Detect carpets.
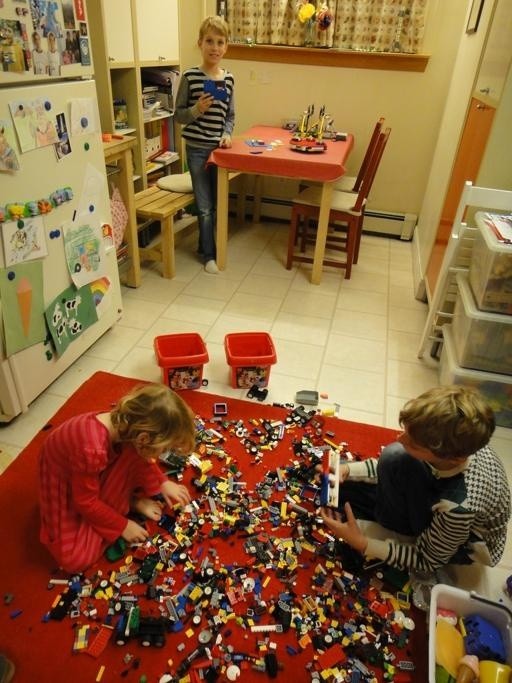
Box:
[2,369,430,683]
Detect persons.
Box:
[30,30,84,76]
[171,13,237,273]
[321,382,511,610]
[34,380,197,571]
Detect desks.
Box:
[205,123,355,286]
[104,133,144,288]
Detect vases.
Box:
[305,20,325,48]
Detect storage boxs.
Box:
[224,331,279,387]
[153,331,210,389]
[470,209,512,313]
[436,320,512,429]
[448,268,512,377]
[426,583,512,683]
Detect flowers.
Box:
[295,0,336,30]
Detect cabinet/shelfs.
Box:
[104,0,183,66]
[414,0,511,339]
[107,63,188,250]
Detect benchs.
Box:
[133,164,249,281]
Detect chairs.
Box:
[292,116,387,255]
[415,180,511,362]
[284,125,393,280]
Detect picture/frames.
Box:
[464,0,485,35]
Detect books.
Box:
[115,238,132,267]
[140,66,180,187]
[105,165,121,177]
